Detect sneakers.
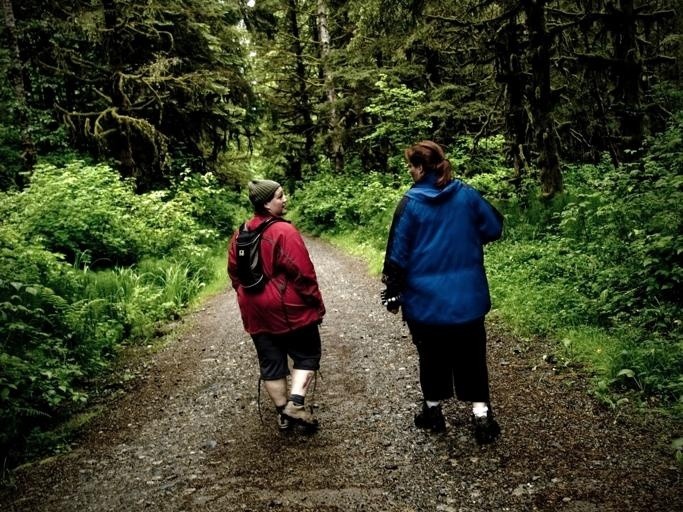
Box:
[276,401,318,431]
[414,399,446,432]
[470,413,499,443]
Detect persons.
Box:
[379,140,505,443]
[224,177,326,435]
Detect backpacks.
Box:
[236,218,274,294]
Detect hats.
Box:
[248,179,280,204]
